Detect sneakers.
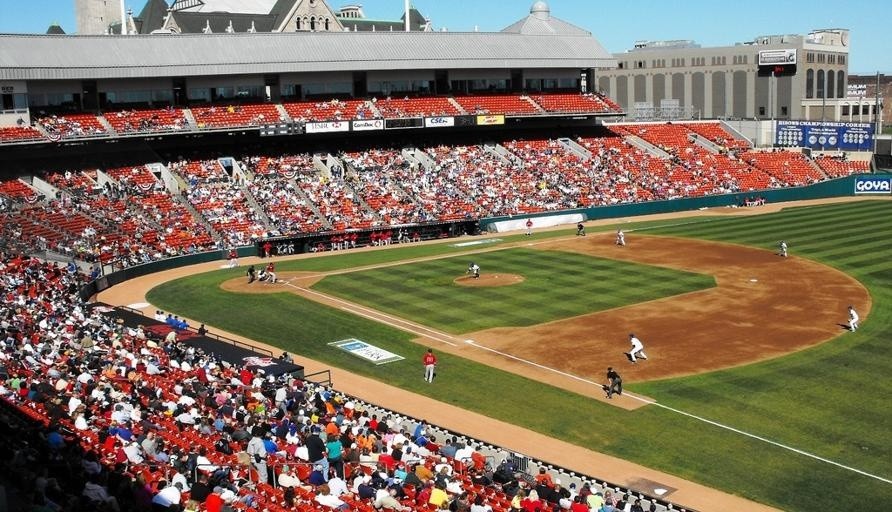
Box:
[424,377,431,383]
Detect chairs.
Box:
[0,108,159,511]
[157,93,850,262]
[161,341,672,512]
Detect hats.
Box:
[428,349,432,352]
[312,427,321,432]
[589,487,597,494]
[175,482,183,489]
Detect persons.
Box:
[266,262,277,285]
[464,261,481,278]
[287,92,875,254]
[775,239,789,260]
[846,306,858,331]
[1,270,214,511]
[626,334,648,364]
[257,267,267,281]
[214,349,656,511]
[1,93,288,269]
[246,263,256,285]
